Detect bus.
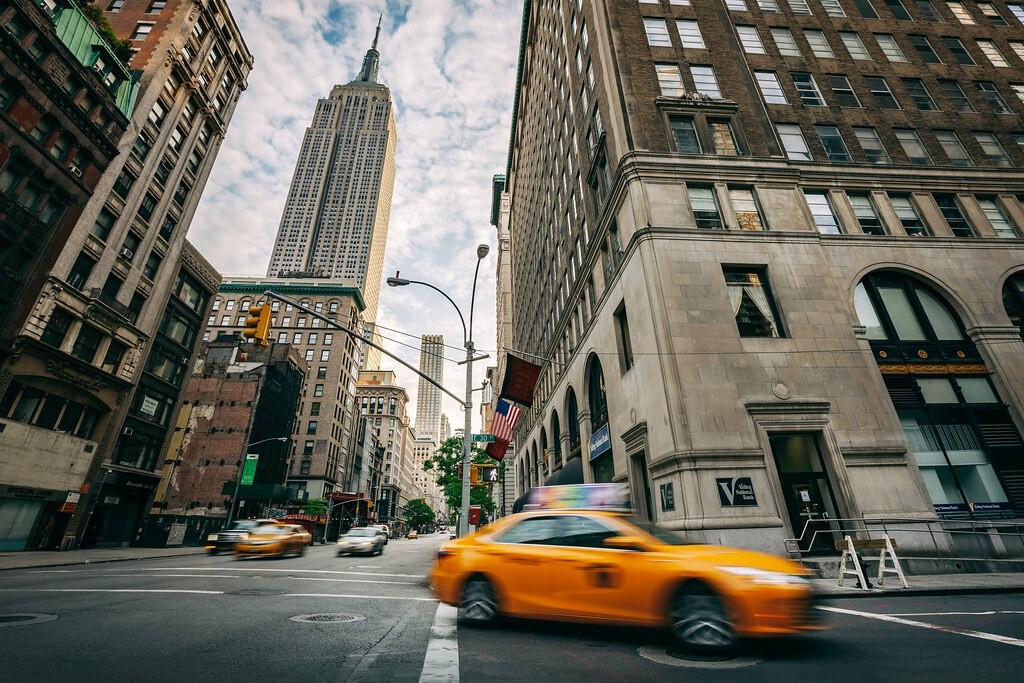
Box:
[438,525,447,535]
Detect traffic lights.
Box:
[368,500,374,508]
[483,465,500,482]
[243,303,270,341]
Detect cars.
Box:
[230,524,313,559]
[449,527,458,539]
[406,529,419,540]
[334,526,385,557]
[367,524,390,545]
[427,482,838,651]
[205,518,279,558]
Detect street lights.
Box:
[226,436,288,529]
[386,242,494,541]
